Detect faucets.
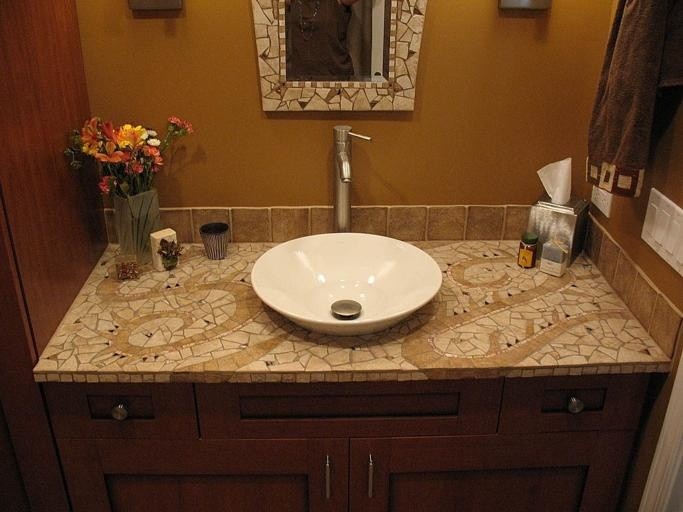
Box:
[331,123,370,232]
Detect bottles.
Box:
[517,232,539,270]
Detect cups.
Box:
[199,222,230,261]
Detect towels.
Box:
[586,0,683,199]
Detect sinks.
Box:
[250,232,443,337]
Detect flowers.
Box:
[67,114,194,195]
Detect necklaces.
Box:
[298,2,320,40]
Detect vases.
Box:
[111,188,158,265]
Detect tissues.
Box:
[526,158,591,267]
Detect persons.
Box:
[284,0,365,82]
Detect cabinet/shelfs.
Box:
[35,362,674,512]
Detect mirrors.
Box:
[250,0,426,110]
[591,186,613,219]
